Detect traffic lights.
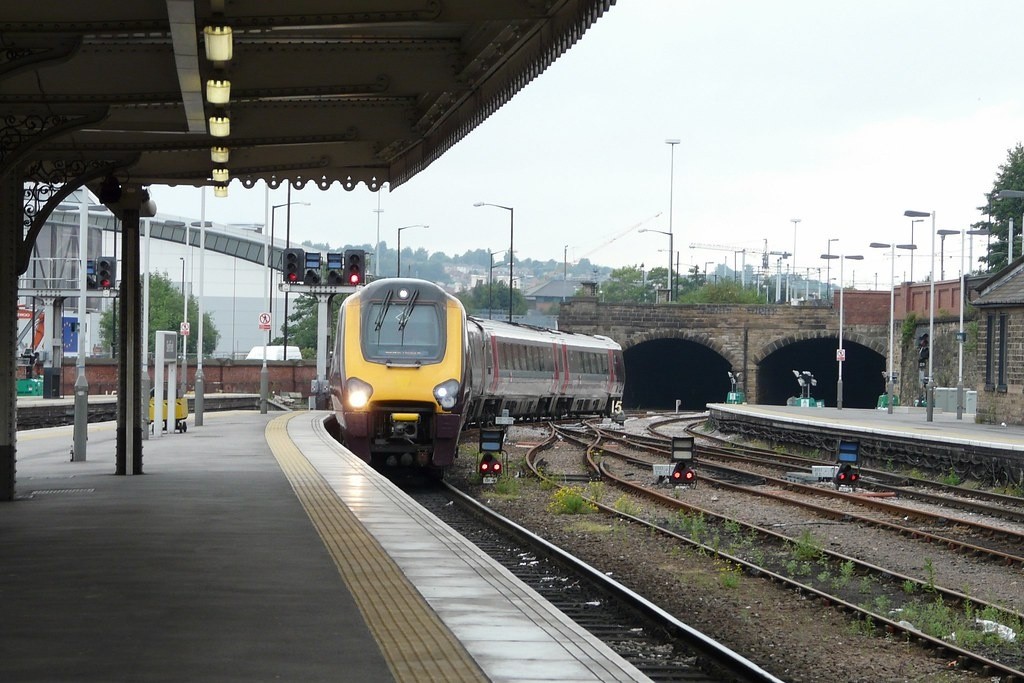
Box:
[282,249,305,283]
[344,250,364,285]
[96,256,116,289]
[478,454,504,475]
[673,466,695,482]
[836,464,860,485]
[919,333,928,363]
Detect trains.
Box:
[325,279,625,487]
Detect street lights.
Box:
[490,250,519,320]
[904,210,934,423]
[871,243,920,413]
[938,190,1023,280]
[474,202,513,322]
[396,225,429,277]
[180,256,186,292]
[791,218,867,408]
[269,202,311,344]
[164,221,215,395]
[639,140,680,301]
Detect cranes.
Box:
[691,238,831,279]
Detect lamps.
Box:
[211,147,228,162]
[214,186,228,197]
[206,80,230,103]
[203,26,232,60]
[212,169,229,181]
[209,117,230,136]
